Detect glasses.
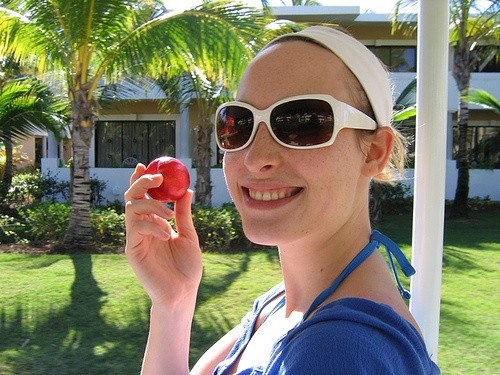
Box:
[215,93,377,152]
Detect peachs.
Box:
[144,156,190,202]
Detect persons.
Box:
[124,23,445,374]
